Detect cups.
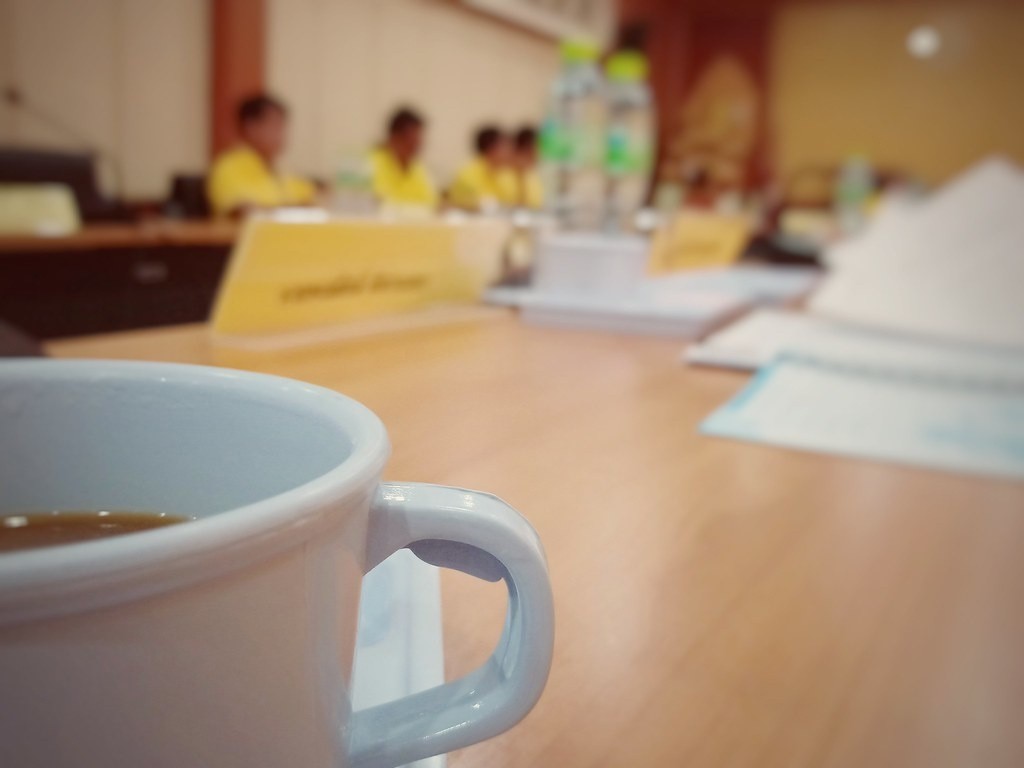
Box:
[0,355,556,768]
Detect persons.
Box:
[205,98,323,219]
[443,128,542,210]
[341,107,446,212]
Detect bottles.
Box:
[540,36,607,230]
[601,52,658,231]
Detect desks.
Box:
[38,297,1024,767]
[0,216,235,341]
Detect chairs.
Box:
[0,144,105,220]
